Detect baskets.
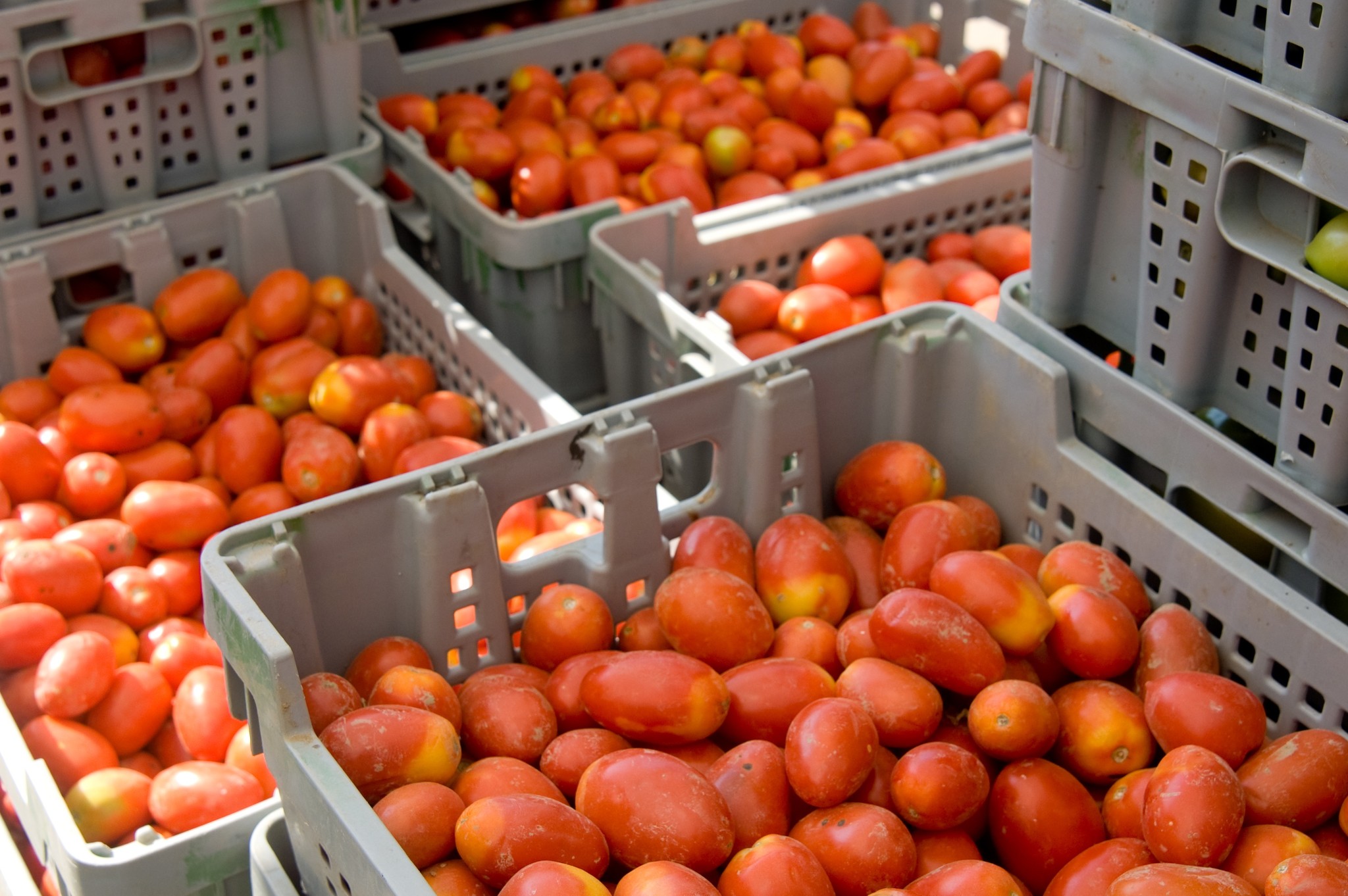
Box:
[2,0,1347,896]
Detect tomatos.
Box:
[304,440,1348,896]
[35,29,231,196]
[377,0,1032,216]
[0,267,648,896]
[1159,158,1348,556]
[716,226,1121,367]
